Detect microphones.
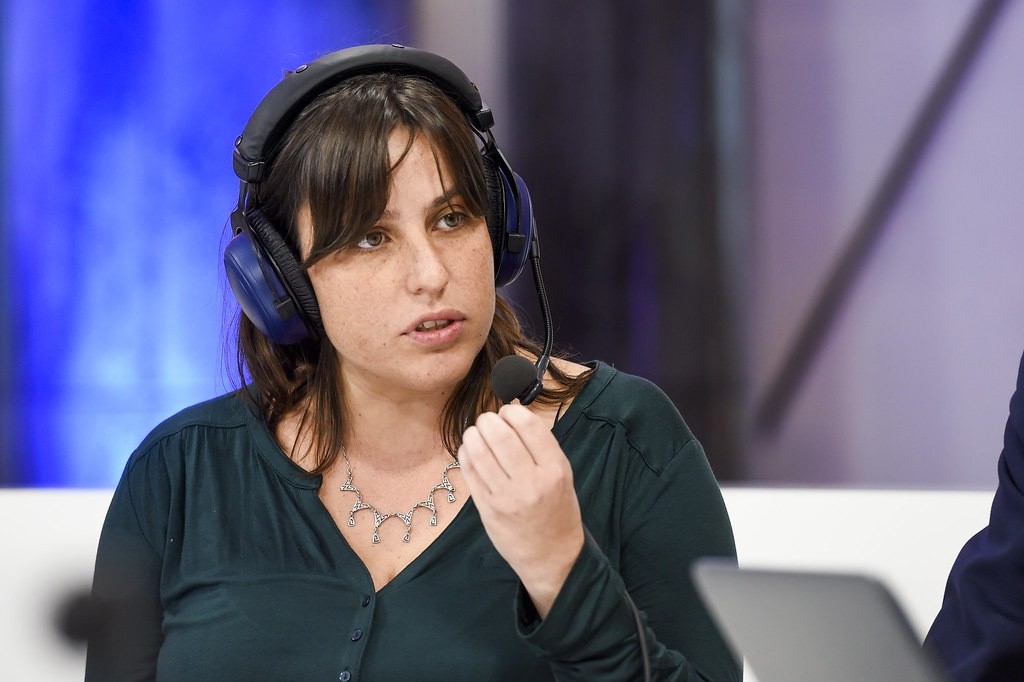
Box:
[490,257,555,405]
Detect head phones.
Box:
[224,42,540,346]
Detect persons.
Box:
[922,348,1024,682]
[85,46,744,682]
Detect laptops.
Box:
[690,563,950,682]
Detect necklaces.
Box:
[339,419,469,544]
